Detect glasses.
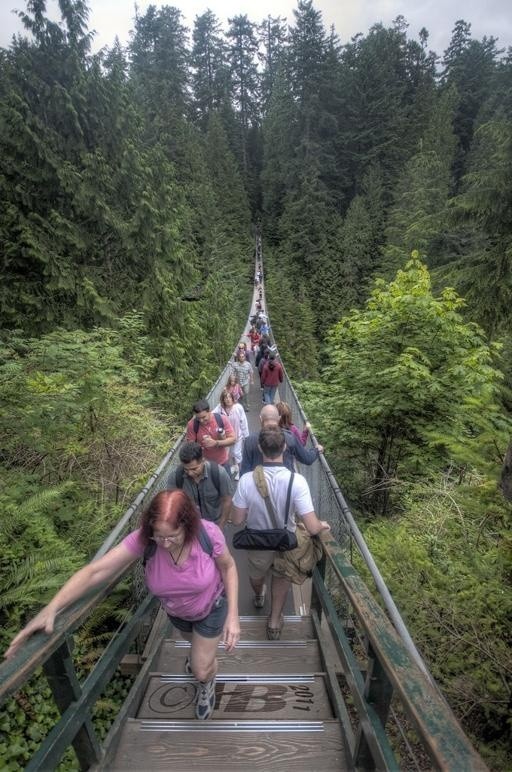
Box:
[146,536,180,543]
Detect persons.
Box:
[186,218,324,477]
[3,489,241,720]
[232,425,331,640]
[166,442,235,534]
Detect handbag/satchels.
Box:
[233,527,298,552]
[276,515,323,586]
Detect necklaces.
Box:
[169,544,184,565]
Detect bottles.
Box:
[216,427,226,440]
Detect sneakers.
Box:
[267,610,285,639]
[253,583,267,608]
[196,677,215,720]
[185,655,192,674]
